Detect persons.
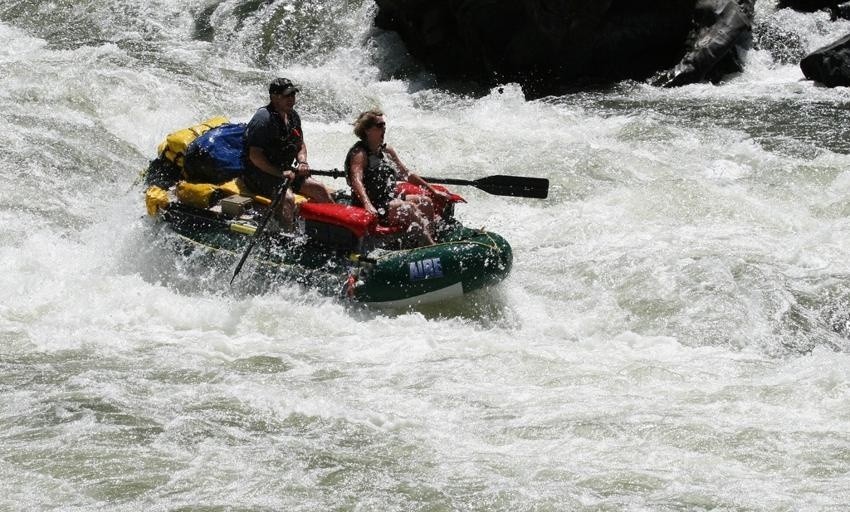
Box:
[344,109,451,246]
[242,77,335,204]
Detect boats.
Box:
[142,156,513,310]
[144,116,513,304]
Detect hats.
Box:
[269,78,300,98]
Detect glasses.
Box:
[283,91,297,98]
[373,121,386,128]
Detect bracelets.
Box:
[300,161,308,165]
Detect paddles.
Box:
[309,168,550,200]
[228,172,296,288]
